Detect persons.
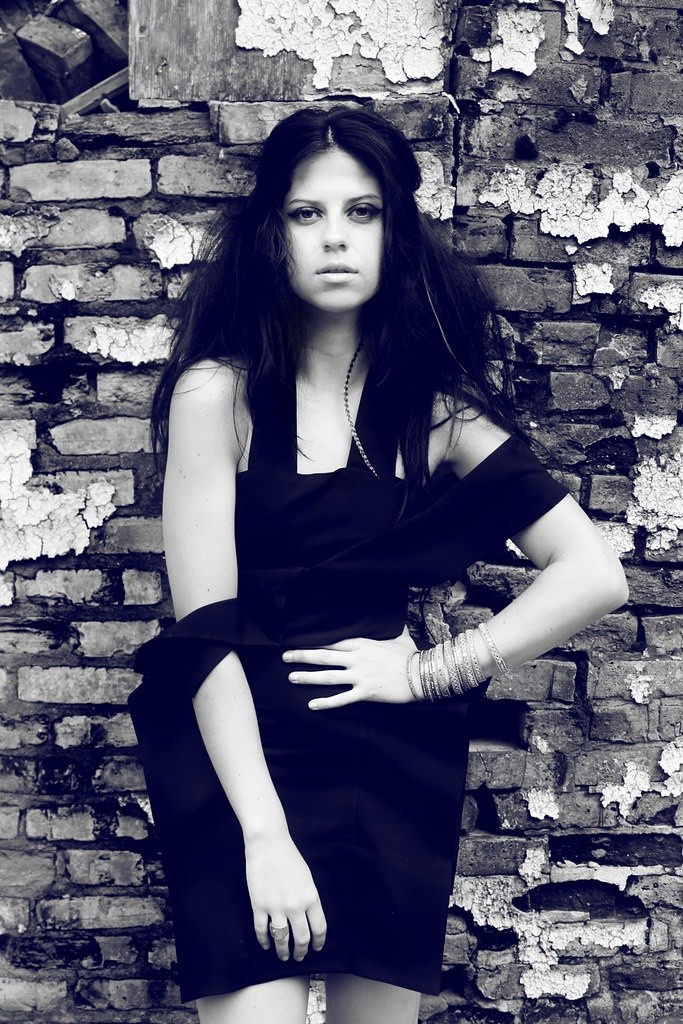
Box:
[128,104,630,1024]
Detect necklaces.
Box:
[343,340,378,476]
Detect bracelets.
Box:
[407,629,486,703]
[478,623,507,674]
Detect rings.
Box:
[269,923,289,941]
